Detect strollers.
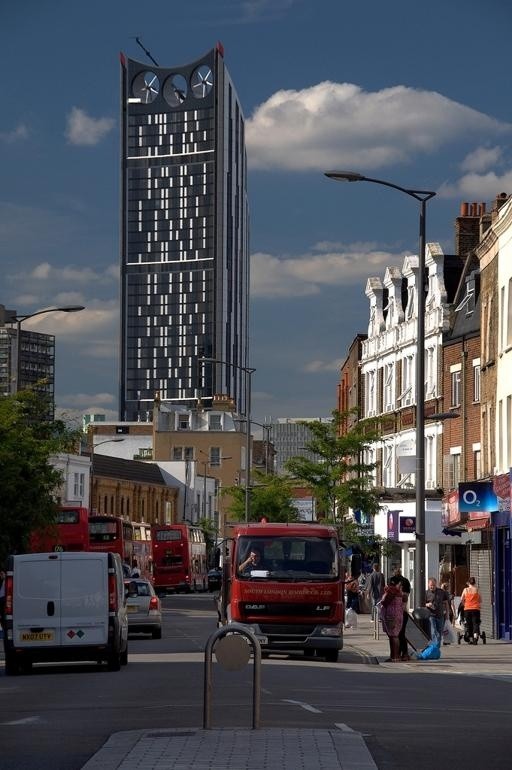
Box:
[456,602,487,646]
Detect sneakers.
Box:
[442,633,479,645]
[385,656,410,662]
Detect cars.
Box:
[122,576,162,640]
[208,567,222,591]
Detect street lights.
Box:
[323,166,460,641]
[198,356,257,522]
[232,418,273,475]
[12,303,85,389]
[89,437,125,513]
[203,456,232,520]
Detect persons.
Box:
[425,578,450,649]
[344,561,412,663]
[460,577,482,646]
[239,546,271,577]
[440,582,457,647]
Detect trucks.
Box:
[6,550,129,677]
[217,517,342,661]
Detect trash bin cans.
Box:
[412,608,433,642]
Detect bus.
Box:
[24,504,209,593]
